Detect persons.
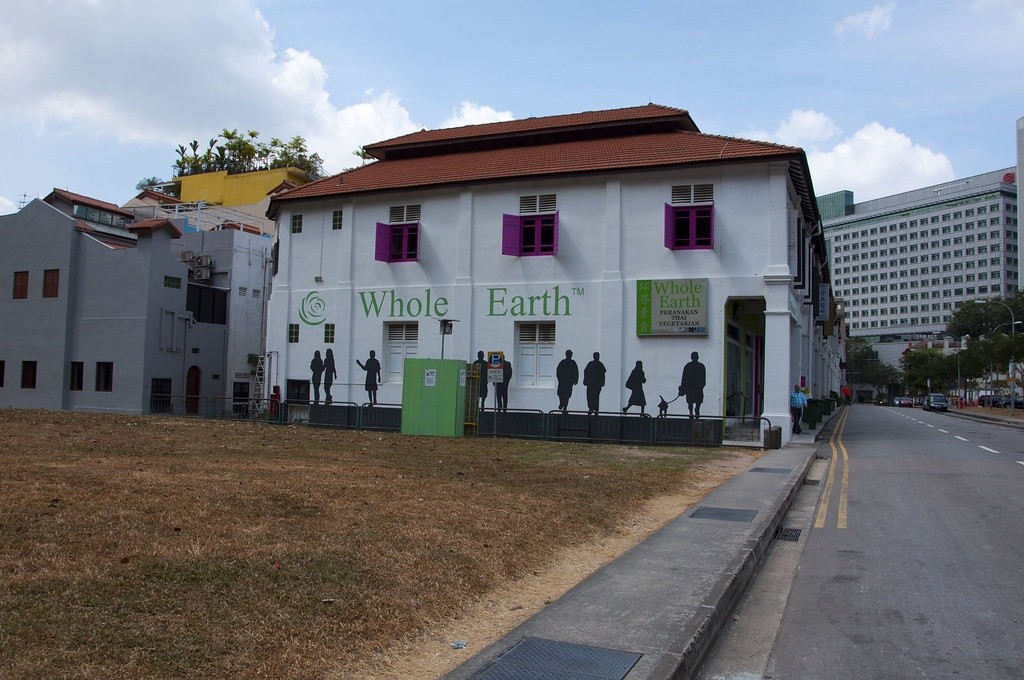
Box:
[790,384,807,434]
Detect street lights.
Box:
[989,320,1022,409]
[909,347,931,393]
[974,296,1023,417]
[932,327,970,408]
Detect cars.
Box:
[893,392,948,413]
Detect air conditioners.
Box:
[195,268,210,279]
[196,254,211,267]
[179,250,193,262]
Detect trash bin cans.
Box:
[824,396,836,412]
[802,398,824,424]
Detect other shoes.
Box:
[792,428,802,434]
